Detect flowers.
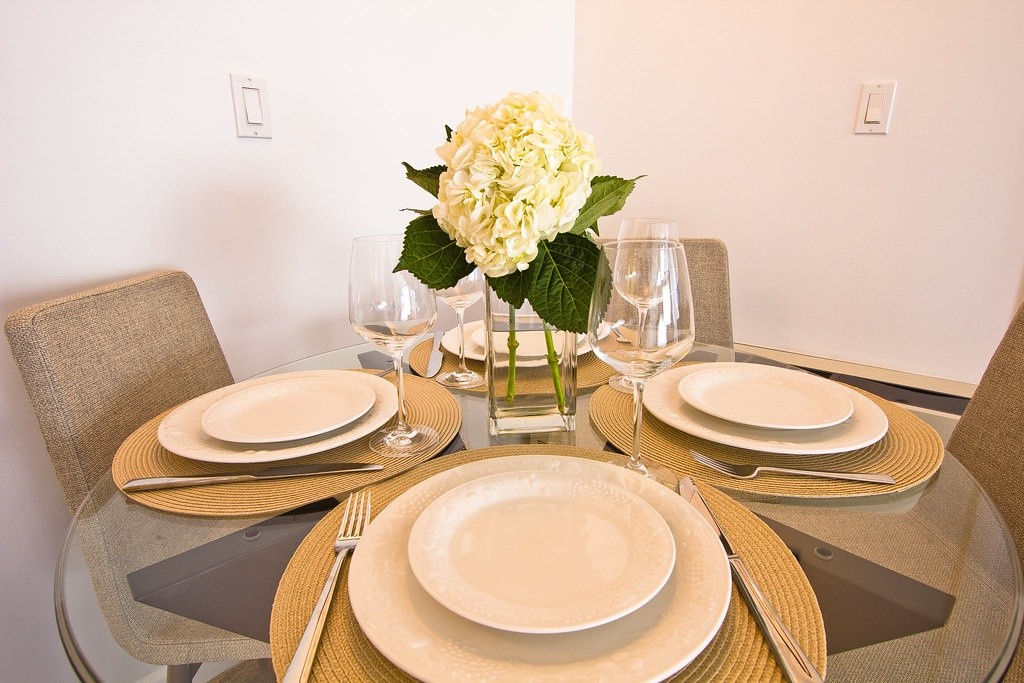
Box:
[390,90,650,416]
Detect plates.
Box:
[201,379,376,443]
[642,362,888,454]
[348,455,732,683]
[472,326,582,356]
[439,319,608,367]
[408,470,677,633]
[678,366,853,430]
[157,370,397,464]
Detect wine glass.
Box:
[428,265,486,389]
[619,218,680,360]
[349,233,439,456]
[588,240,695,492]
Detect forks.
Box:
[612,323,633,343]
[282,489,373,683]
[689,447,896,485]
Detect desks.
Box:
[51,328,1024,682]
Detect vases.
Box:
[485,270,581,437]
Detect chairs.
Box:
[529,238,739,446]
[3,268,277,681]
[729,299,1024,682]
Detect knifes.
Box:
[122,463,383,492]
[426,329,443,378]
[678,472,825,683]
